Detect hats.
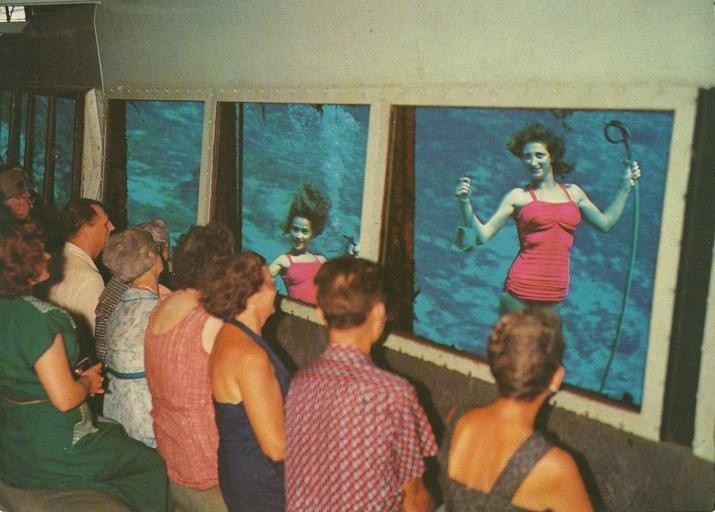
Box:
[0,169,40,202]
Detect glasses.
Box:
[18,194,38,204]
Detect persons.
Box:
[202,253,293,512]
[144,223,227,511]
[0,164,32,233]
[45,198,114,334]
[283,255,439,511]
[92,225,167,360]
[102,231,165,448]
[453,123,641,315]
[435,309,597,512]
[0,224,169,511]
[269,182,358,303]
